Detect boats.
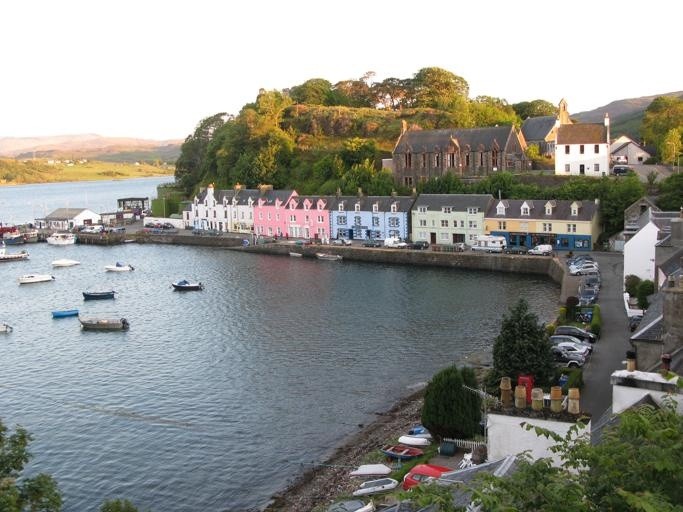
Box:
[329,428,435,512]
[51,309,79,319]
[105,263,135,271]
[46,233,76,245]
[0,225,39,245]
[1,324,12,332]
[315,251,344,262]
[171,280,203,291]
[19,273,55,283]
[77,317,130,331]
[51,259,80,267]
[289,252,302,257]
[83,290,117,301]
[0,244,29,262]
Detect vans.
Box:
[613,156,628,164]
[614,165,634,174]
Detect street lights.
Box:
[665,142,676,170]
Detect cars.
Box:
[192,228,224,236]
[546,253,601,366]
[68,223,126,234]
[331,235,554,255]
[145,221,174,229]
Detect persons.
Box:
[280,232,283,239]
[286,232,288,239]
[275,233,277,239]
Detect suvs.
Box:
[402,463,454,491]
[629,315,641,333]
